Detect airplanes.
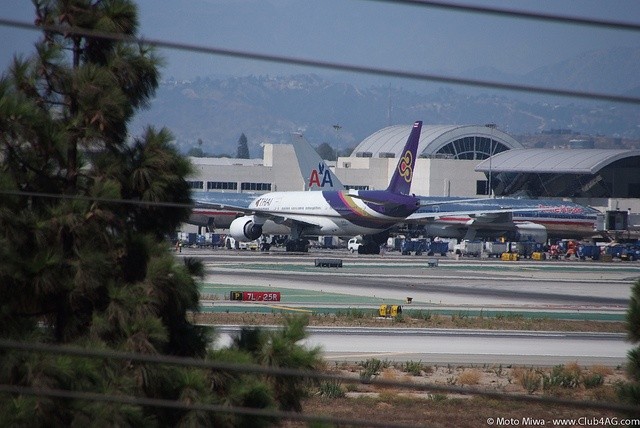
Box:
[188,133,604,248]
[184,120,559,255]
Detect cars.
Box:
[225,234,258,250]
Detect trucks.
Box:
[347,237,363,253]
